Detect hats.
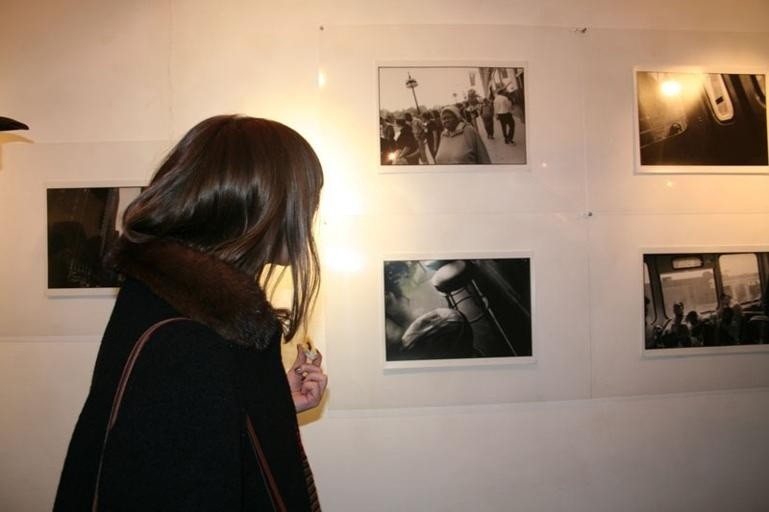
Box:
[441,106,462,120]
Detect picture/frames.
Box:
[633,64,769,176]
[638,243,768,358]
[373,59,534,174]
[41,177,153,296]
[379,252,538,368]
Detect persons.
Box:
[380,85,527,164]
[645,288,761,351]
[52,115,327,512]
